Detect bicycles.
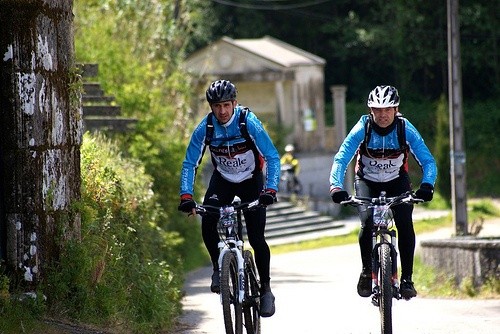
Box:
[339,189,434,334]
[277,155,303,195]
[178,197,267,334]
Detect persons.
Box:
[178,80,280,318]
[329,85,437,298]
[280,144,300,195]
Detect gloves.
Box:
[332,190,349,207]
[259,189,276,205]
[415,187,433,203]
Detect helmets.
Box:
[206,80,236,102]
[367,85,400,108]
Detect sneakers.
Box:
[400,279,416,296]
[357,273,372,297]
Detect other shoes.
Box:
[260,291,275,317]
[210,274,220,293]
